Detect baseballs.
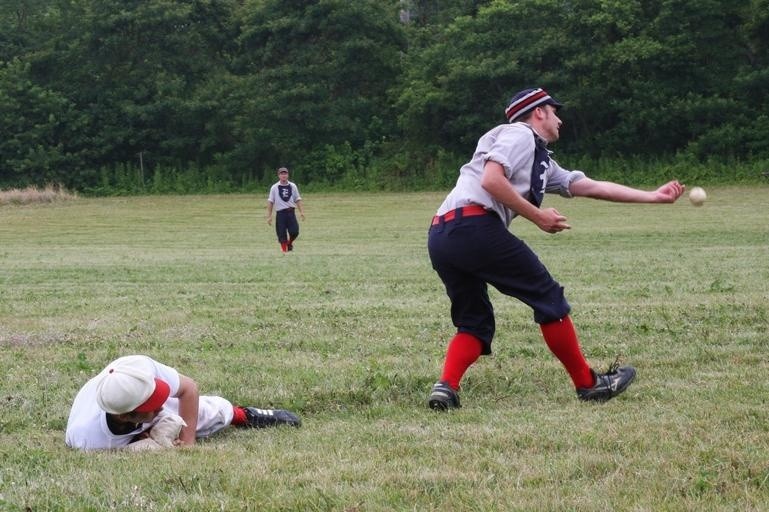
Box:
[689,185,707,206]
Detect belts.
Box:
[430,205,487,227]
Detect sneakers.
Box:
[429,379,461,410]
[576,366,636,408]
[236,406,303,431]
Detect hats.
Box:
[96,366,170,416]
[277,167,288,174]
[503,87,566,123]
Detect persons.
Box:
[65,352,304,451]
[266,166,306,251]
[427,87,687,411]
